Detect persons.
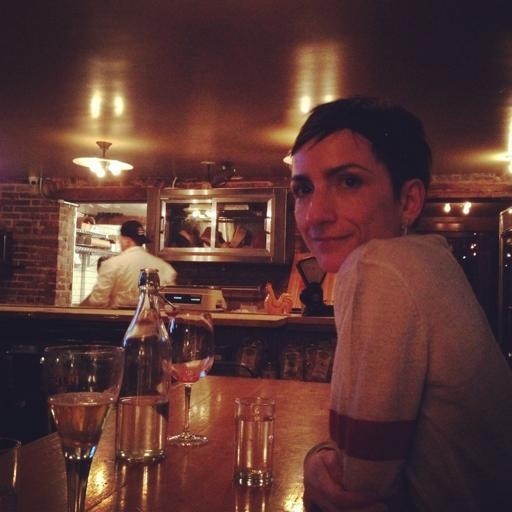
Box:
[80,220,177,306]
[289,97,512,512]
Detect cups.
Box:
[233,396,277,488]
[0,437,26,512]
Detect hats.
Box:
[120,220,151,244]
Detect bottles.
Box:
[111,269,174,466]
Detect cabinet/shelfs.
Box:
[145,186,296,265]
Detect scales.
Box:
[158,285,228,312]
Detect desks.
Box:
[2,371,333,512]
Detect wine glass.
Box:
[41,341,124,512]
[164,309,215,449]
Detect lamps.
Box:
[72,140,134,178]
